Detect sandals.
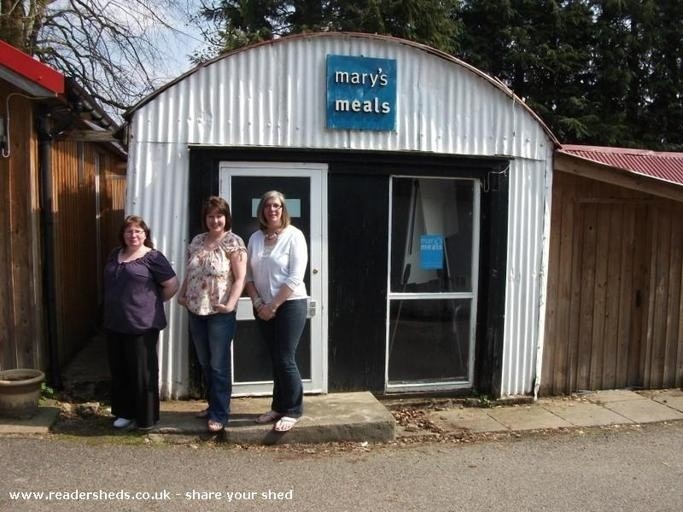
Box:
[195,407,224,433]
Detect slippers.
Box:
[275,416,302,433]
[255,410,281,424]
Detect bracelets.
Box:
[253,296,264,312]
[268,301,277,313]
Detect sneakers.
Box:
[114,417,132,428]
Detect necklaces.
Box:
[264,229,279,240]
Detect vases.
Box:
[0,368,47,421]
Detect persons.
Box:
[245,191,309,434]
[99,214,179,431]
[178,195,248,433]
